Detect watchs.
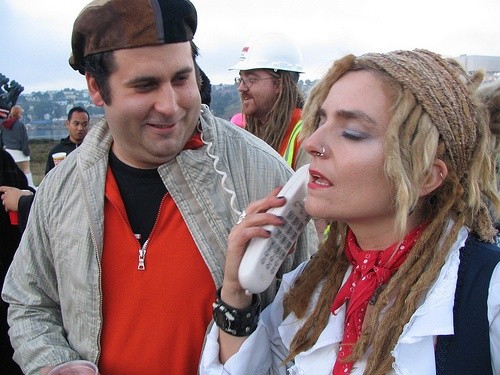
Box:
[211,287,262,337]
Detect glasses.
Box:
[234,76,280,88]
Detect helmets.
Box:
[228,38,304,75]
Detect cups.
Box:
[48,361,98,375]
[52,152,66,167]
[5,190,30,224]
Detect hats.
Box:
[69,0,197,75]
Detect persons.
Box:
[0,0,500,375]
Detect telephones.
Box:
[236,163,312,294]
